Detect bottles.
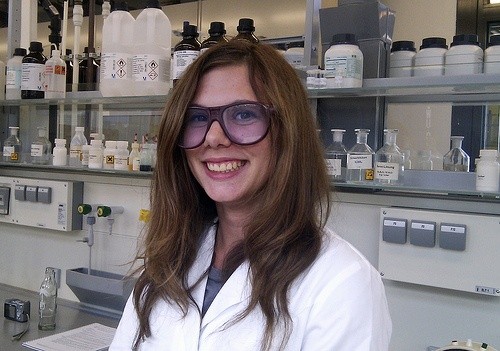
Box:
[346,129,375,186]
[388,33,500,78]
[443,136,469,173]
[37,267,58,331]
[2,125,156,172]
[170,18,260,92]
[0,41,96,100]
[323,33,363,89]
[476,149,500,194]
[99,0,173,97]
[276,42,305,70]
[323,128,348,183]
[373,129,405,187]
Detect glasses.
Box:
[175,101,276,148]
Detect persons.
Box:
[109,40,392,351]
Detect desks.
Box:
[0,289,121,351]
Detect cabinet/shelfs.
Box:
[0,73,500,199]
